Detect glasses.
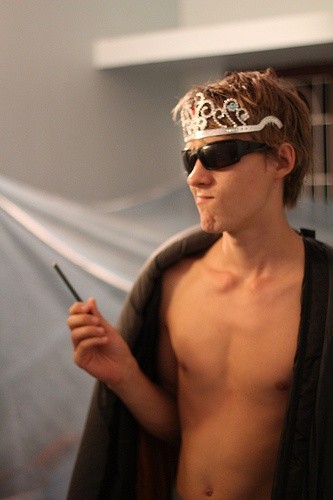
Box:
[182,138,268,176]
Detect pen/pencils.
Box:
[52,262,93,320]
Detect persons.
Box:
[67,68,333,500]
